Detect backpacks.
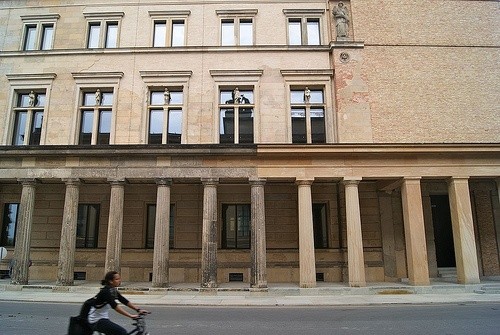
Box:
[80,297,95,316]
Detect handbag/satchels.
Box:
[68,315,93,335]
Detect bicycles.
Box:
[97,311,151,335]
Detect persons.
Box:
[234,88,241,105]
[28,90,35,107]
[95,88,102,105]
[87,271,149,335]
[332,1,350,38]
[163,88,171,105]
[304,87,311,102]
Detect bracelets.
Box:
[136,308,141,313]
